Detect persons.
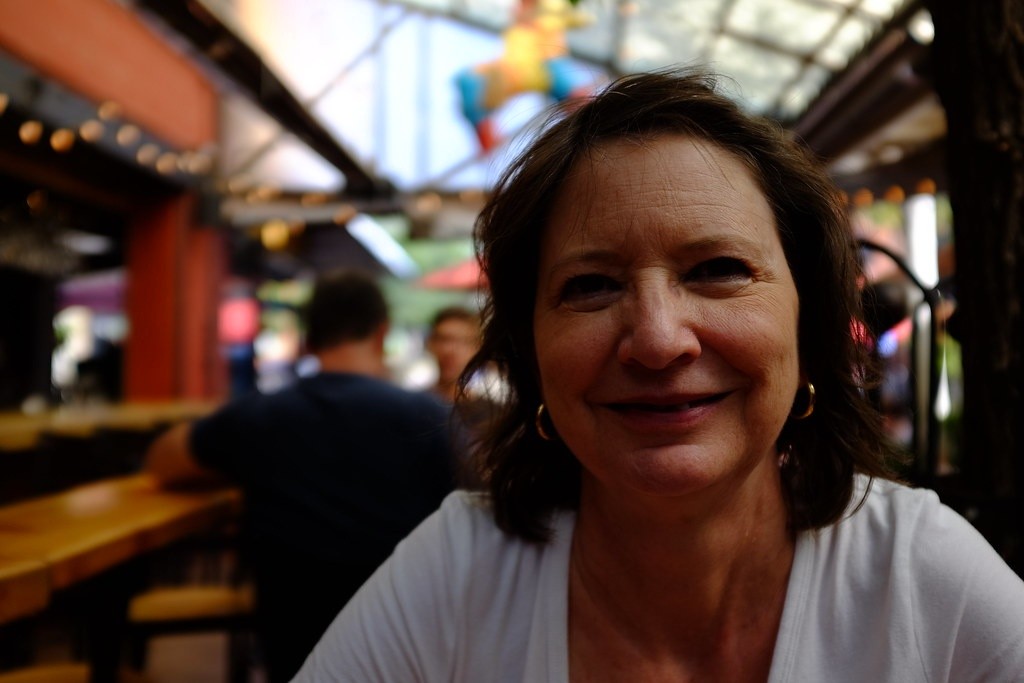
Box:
[149,265,468,683]
[416,310,510,492]
[291,67,1024,682]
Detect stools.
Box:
[127,583,255,683]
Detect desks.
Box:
[0,475,247,683]
